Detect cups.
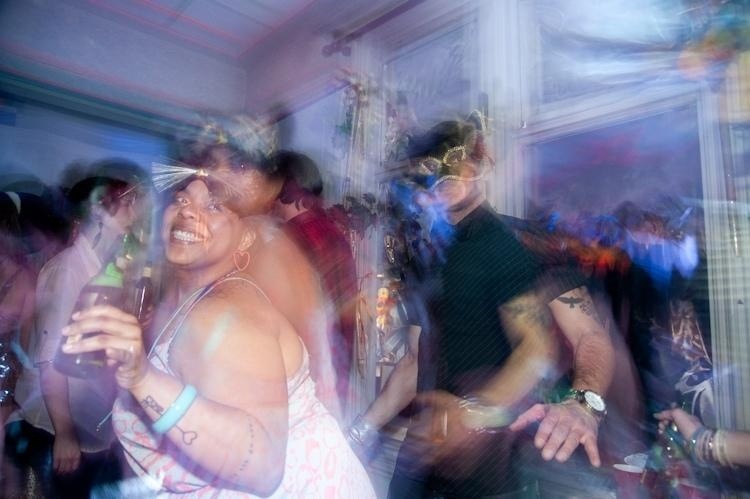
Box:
[612,463,643,499]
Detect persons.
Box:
[4,115,749,498]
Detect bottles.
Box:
[132,260,153,322]
[639,397,692,496]
[53,224,138,379]
